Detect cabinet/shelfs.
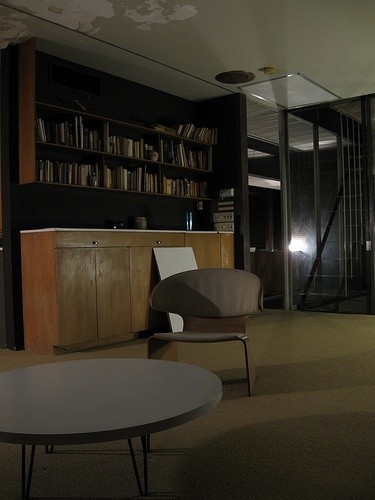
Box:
[18,37,214,201]
[19,227,234,355]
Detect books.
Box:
[33,109,218,200]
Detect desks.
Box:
[0,358,222,500]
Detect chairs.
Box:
[147,268,264,397]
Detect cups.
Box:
[147,151,159,162]
